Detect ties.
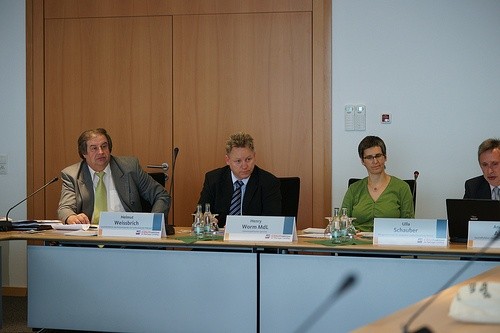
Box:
[229,180,244,215]
[93,172,107,224]
[494,188,500,200]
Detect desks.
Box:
[0,222,500,333]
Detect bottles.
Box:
[339,208,349,242]
[333,208,341,243]
[192,214,197,239]
[203,203,215,240]
[194,205,203,240]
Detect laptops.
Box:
[445,199,500,243]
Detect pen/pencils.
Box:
[89,226,97,229]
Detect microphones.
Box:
[409,171,419,219]
[164,148,179,235]
[0,177,58,231]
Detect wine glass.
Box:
[348,217,357,240]
[324,216,334,240]
[212,214,219,236]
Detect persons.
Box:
[192,133,281,228]
[58,128,172,225]
[339,135,415,232]
[462,139,500,200]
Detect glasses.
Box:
[364,154,382,160]
